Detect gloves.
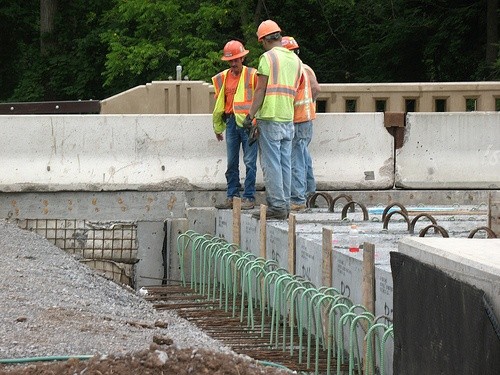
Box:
[247,127,257,147]
[243,114,253,133]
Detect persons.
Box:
[242,19,303,219]
[280,36,320,210]
[212,40,259,209]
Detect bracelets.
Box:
[246,114,251,120]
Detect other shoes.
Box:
[241,197,255,210]
[214,198,236,209]
[252,206,291,220]
[289,199,320,211]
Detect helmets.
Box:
[257,20,282,40]
[282,36,299,50]
[221,40,249,62]
